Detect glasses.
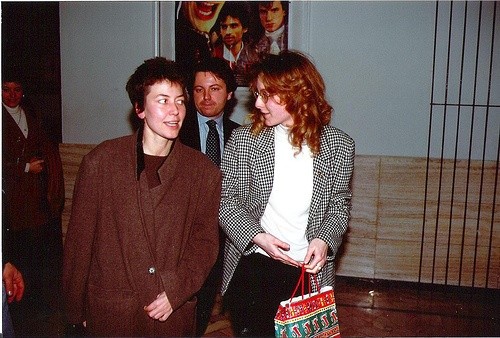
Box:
[253,86,274,103]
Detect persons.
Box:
[176,1,288,87]
[62,57,224,338]
[179,60,242,168]
[219,50,355,338]
[1,248,23,338]
[2,75,61,312]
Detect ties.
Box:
[207,119,221,165]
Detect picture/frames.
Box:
[154,0,309,102]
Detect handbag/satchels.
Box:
[274,262,341,338]
